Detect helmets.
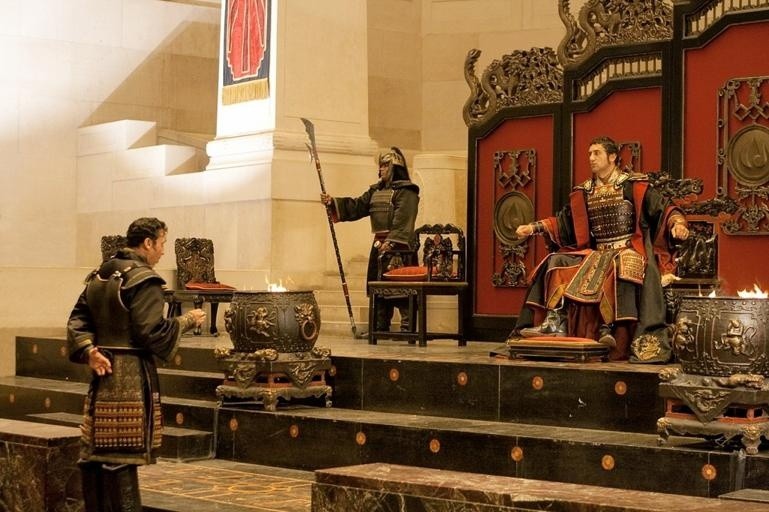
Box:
[378,146,406,179]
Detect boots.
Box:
[519,309,567,338]
[597,320,617,348]
[399,306,410,332]
[356,298,392,341]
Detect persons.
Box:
[66,216,208,510]
[514,136,690,350]
[320,147,421,343]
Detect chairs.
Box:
[100,235,239,338]
[365,224,469,348]
[506,169,739,363]
